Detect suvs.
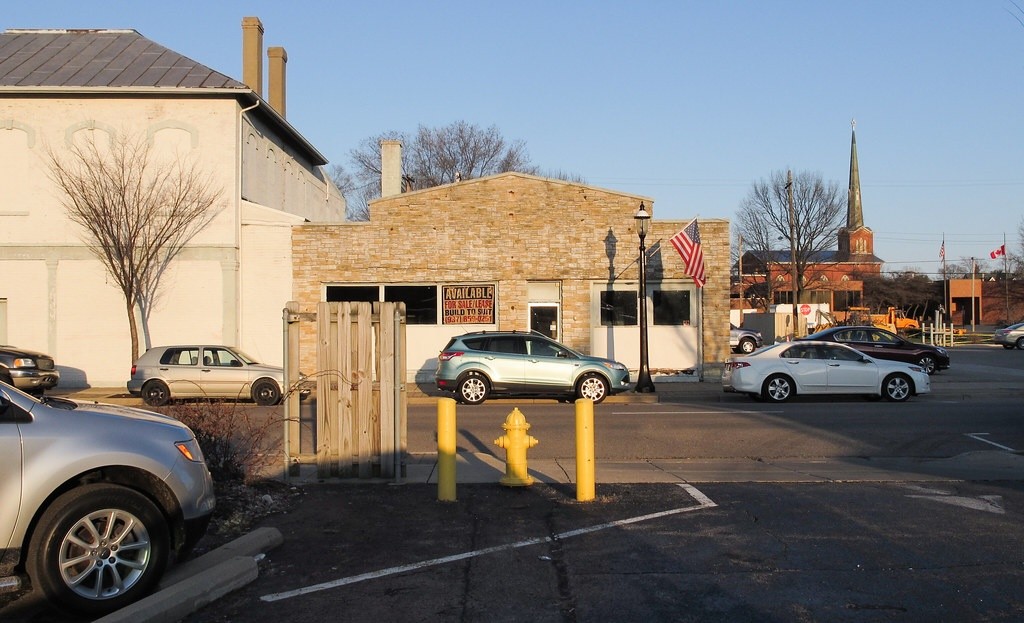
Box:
[126,345,312,406]
[433,329,631,405]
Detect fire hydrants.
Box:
[495,406,539,487]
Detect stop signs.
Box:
[800,304,812,315]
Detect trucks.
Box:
[860,306,920,335]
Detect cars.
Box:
[0,377,217,618]
[1,347,62,393]
[993,322,1024,352]
[730,340,931,402]
[720,341,857,401]
[730,322,764,354]
[790,326,951,375]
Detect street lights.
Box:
[634,199,656,392]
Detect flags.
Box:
[990,244,1005,259]
[669,216,706,288]
[939,241,945,263]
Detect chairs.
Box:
[852,331,866,341]
[203,356,211,365]
[191,358,197,365]
